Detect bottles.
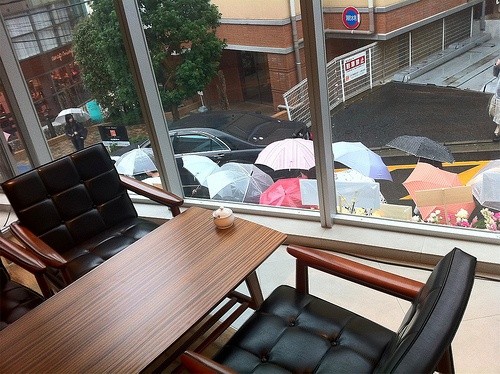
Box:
[212,206,234,229]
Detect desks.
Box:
[0,204,290,374]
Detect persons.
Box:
[487,58,500,141]
[65,114,84,151]
[144,166,159,178]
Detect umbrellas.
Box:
[114,135,500,226]
[53,108,91,126]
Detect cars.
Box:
[109,108,315,199]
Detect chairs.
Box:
[0,232,56,333]
[0,142,188,296]
[176,241,478,374]
